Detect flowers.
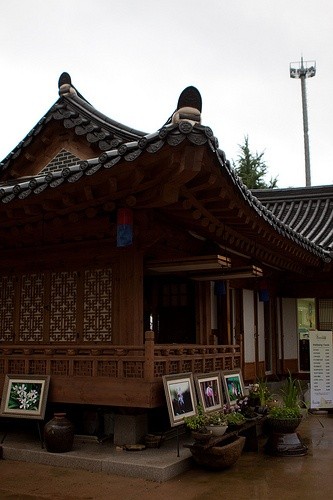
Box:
[224,396,249,413]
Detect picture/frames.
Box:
[194,372,224,414]
[221,368,245,407]
[0,374,50,420]
[162,372,198,428]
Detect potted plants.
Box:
[267,407,303,433]
[258,379,268,414]
[205,414,228,436]
[249,384,260,406]
[226,413,246,429]
[183,405,212,440]
